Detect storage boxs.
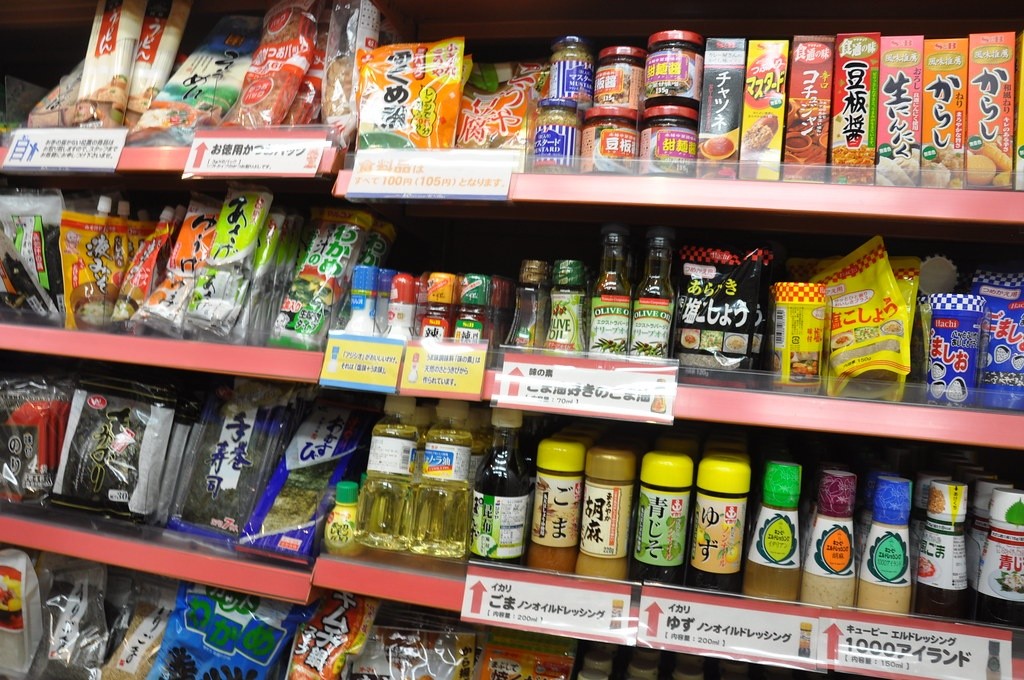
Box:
[921,38,969,189]
[874,35,924,187]
[830,32,881,187]
[695,38,747,179]
[783,35,836,183]
[966,32,1016,191]
[1013,30,1024,191]
[738,40,790,180]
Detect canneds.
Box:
[530,31,704,178]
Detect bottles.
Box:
[801,466,857,610]
[504,255,593,353]
[742,460,803,604]
[910,447,1024,634]
[588,231,633,355]
[321,395,547,567]
[855,468,916,616]
[528,430,637,582]
[630,234,677,359]
[631,421,752,599]
[342,262,511,363]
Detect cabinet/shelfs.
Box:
[0,0,1024,680]
